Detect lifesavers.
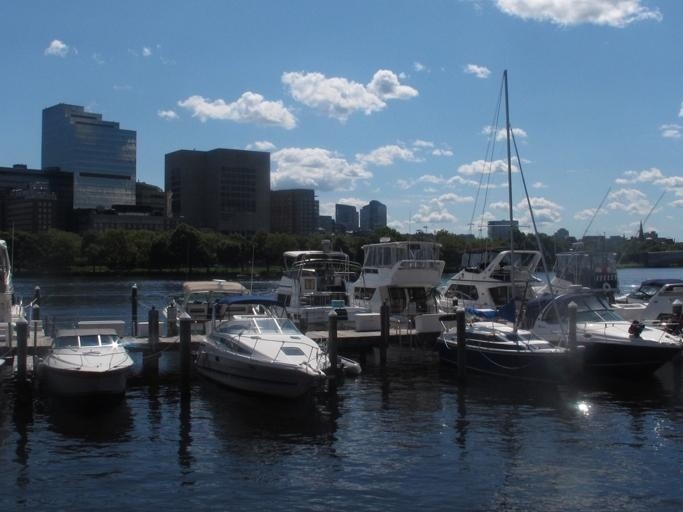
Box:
[603,282,611,289]
